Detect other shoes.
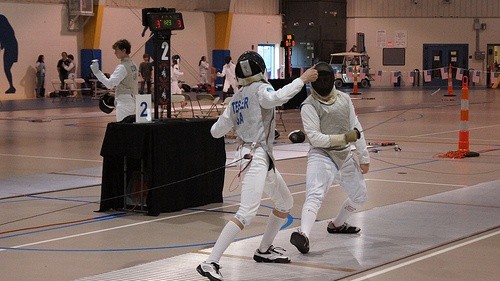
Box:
[221,102,224,105]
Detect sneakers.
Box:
[253,245,291,263]
[196,261,224,281]
[327,221,361,234]
[290,228,310,254]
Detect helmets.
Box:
[225,55,232,64]
[309,62,338,105]
[235,50,268,86]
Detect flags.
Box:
[391,72,397,83]
[424,71,431,82]
[491,73,497,83]
[346,67,353,78]
[408,72,414,83]
[375,71,382,80]
[456,68,463,80]
[472,72,479,83]
[360,69,366,78]
[441,68,448,80]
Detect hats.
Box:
[98,93,115,114]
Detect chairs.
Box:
[276,107,286,132]
[172,92,237,139]
[51,79,91,103]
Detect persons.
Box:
[199,55,209,86]
[172,55,184,95]
[139,54,152,95]
[57,51,70,89]
[290,61,370,253]
[90,39,138,124]
[63,54,76,94]
[36,54,46,98]
[217,56,240,105]
[196,50,318,281]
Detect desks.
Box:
[89,79,100,100]
[98,117,227,215]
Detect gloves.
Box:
[90,62,99,74]
[345,130,357,143]
